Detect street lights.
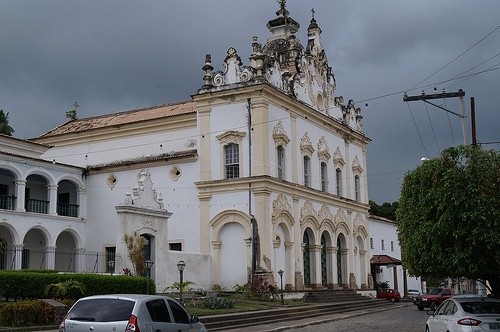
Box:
[277,270,285,303]
[109,260,115,276]
[176,261,185,303]
[144,259,154,293]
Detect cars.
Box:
[58,293,208,332]
[424,296,500,332]
[407,289,420,298]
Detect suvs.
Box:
[413,288,454,312]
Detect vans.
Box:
[377,288,402,303]
